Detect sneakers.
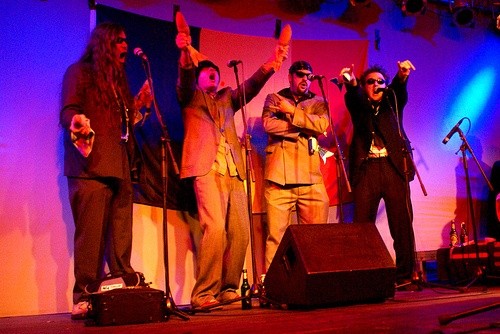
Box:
[71,301,92,319]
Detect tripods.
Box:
[142,60,288,320]
[383,90,500,292]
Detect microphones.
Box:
[442,118,464,144]
[134,47,149,62]
[227,60,242,67]
[308,74,325,81]
[374,86,393,93]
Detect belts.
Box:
[367,153,389,158]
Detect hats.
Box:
[195,60,221,84]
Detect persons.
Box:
[175,32,288,313]
[342,60,416,288]
[262,60,329,278]
[59,21,153,319]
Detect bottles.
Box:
[259,274,270,308]
[450,220,458,247]
[460,222,469,244]
[240,269,252,309]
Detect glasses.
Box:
[295,71,311,78]
[366,78,386,85]
[116,37,128,43]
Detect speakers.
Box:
[264,221,395,309]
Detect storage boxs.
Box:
[437,242,500,283]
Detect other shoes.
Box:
[215,290,241,304]
[193,295,220,311]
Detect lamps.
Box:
[350,0,500,35]
